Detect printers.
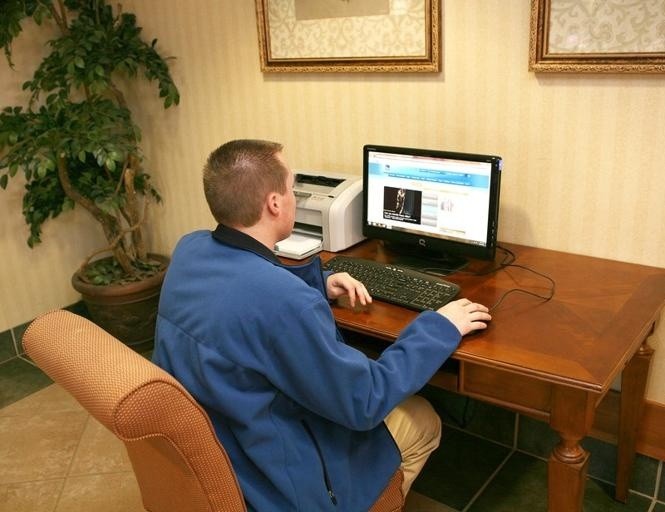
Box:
[273,169,368,261]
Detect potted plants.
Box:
[0,0,180,353]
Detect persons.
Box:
[149,137,495,512]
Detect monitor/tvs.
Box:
[362,145,502,277]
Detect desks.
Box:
[277,236,665,512]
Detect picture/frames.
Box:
[528,0,665,73]
[255,0,442,73]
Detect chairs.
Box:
[22,310,405,512]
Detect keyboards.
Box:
[321,255,461,312]
